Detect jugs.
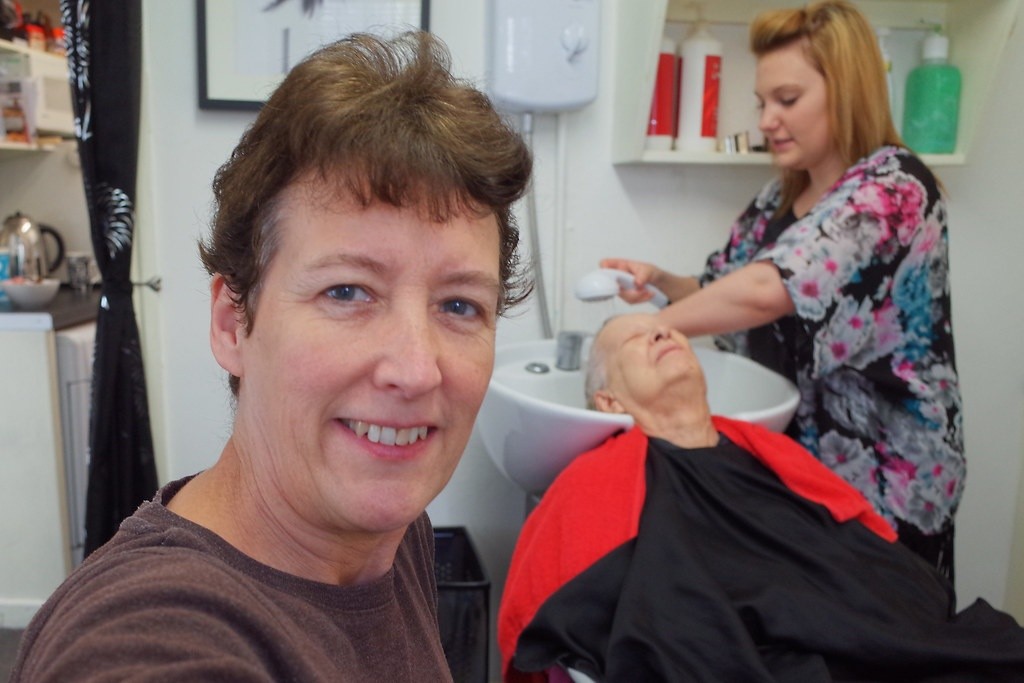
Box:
[0,212,65,278]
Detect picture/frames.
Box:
[196,0,431,112]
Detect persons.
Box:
[598,0,966,584]
[497,313,1024,683]
[8,28,531,683]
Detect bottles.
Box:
[675,26,724,153]
[645,34,676,150]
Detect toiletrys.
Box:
[675,0,724,153]
[875,23,896,116]
[901,18,964,154]
[646,34,676,160]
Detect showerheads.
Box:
[574,267,669,308]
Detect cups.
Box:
[66,253,92,295]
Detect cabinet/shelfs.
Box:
[0,1,78,152]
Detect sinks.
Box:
[477,341,801,496]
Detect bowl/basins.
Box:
[4,279,61,307]
